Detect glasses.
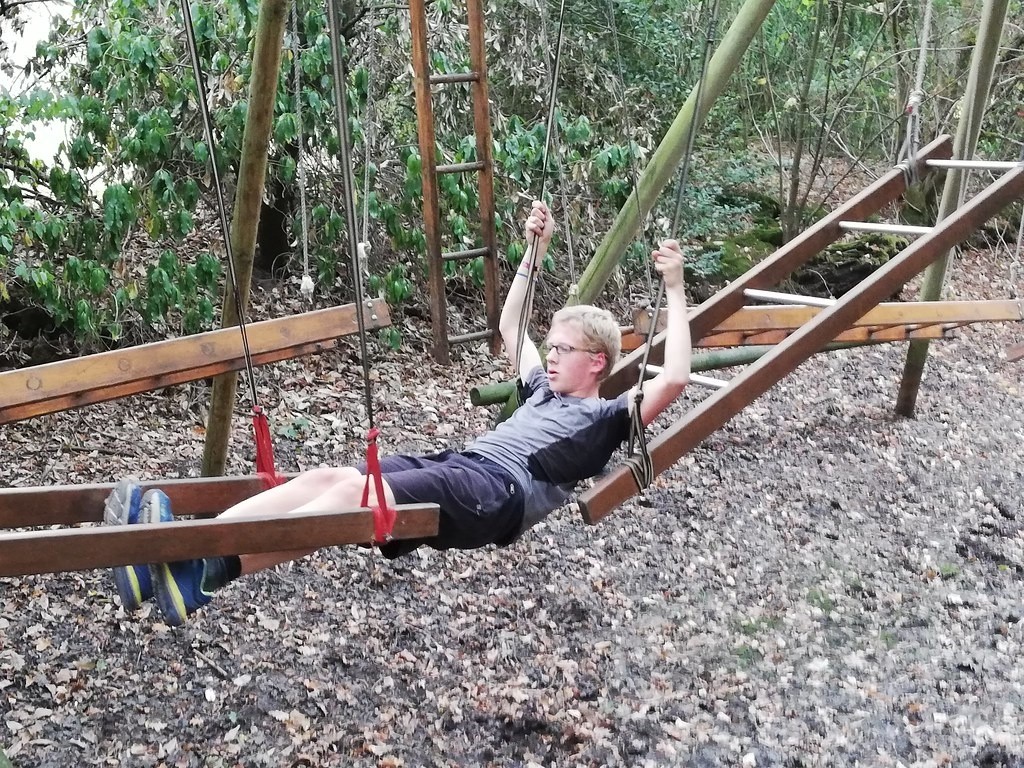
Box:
[543,343,609,359]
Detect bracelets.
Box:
[520,261,540,272]
[516,273,538,283]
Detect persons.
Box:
[103,197,692,626]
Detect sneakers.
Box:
[103,475,154,612]
[138,489,217,626]
